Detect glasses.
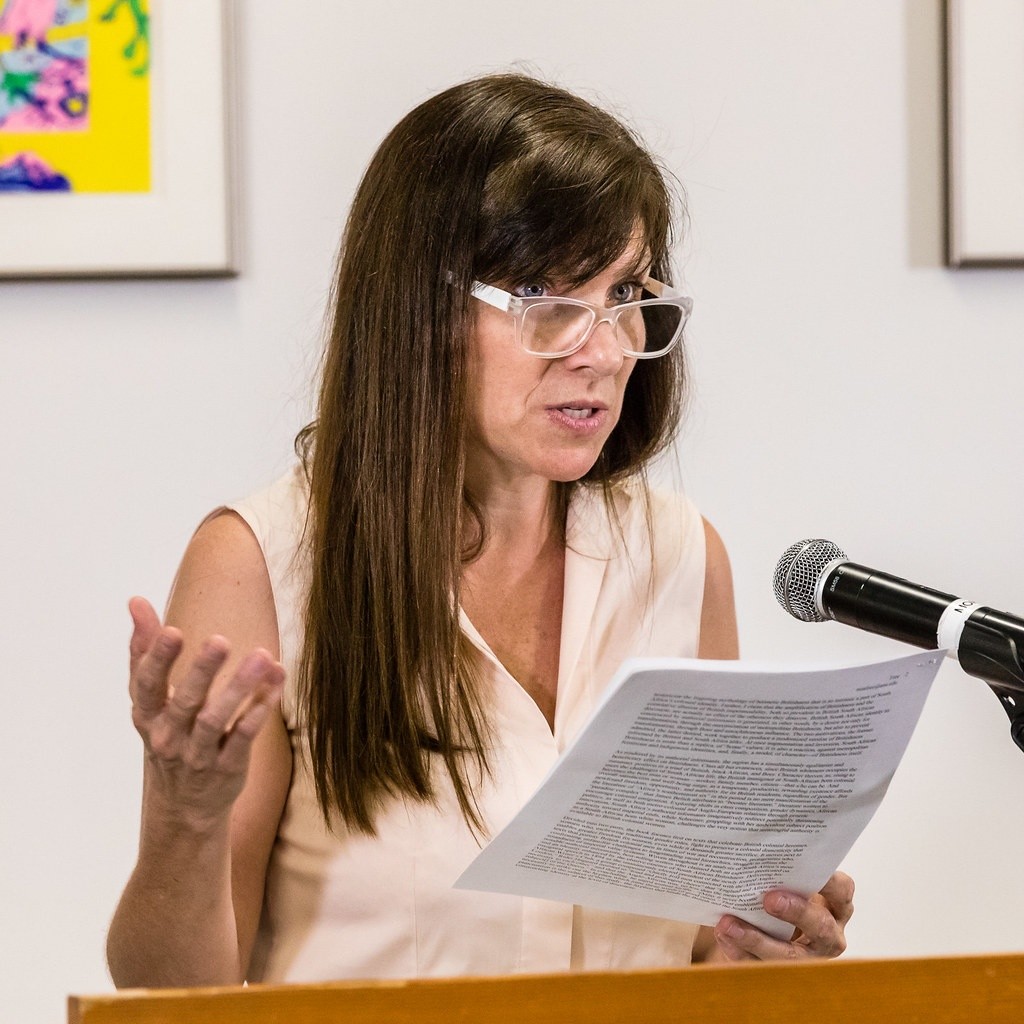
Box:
[442,269,696,359]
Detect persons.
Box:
[107,71,855,990]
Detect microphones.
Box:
[772,538,1024,694]
